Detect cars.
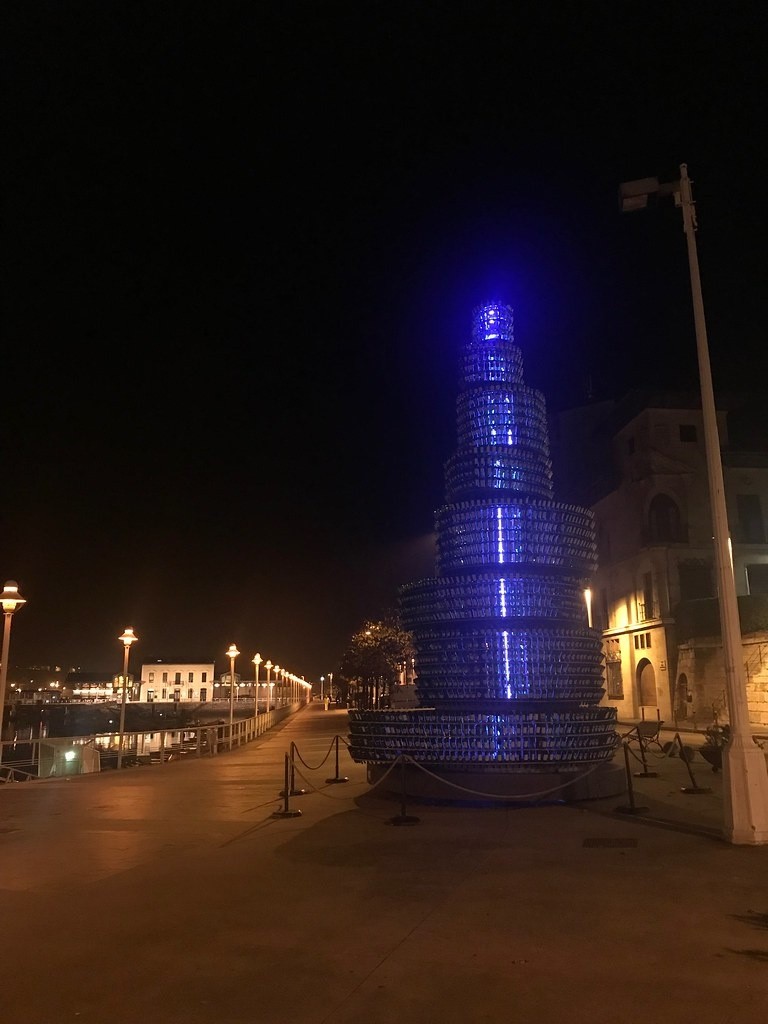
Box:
[356,692,373,707]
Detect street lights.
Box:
[118,627,138,768]
[226,643,240,751]
[264,660,273,712]
[251,653,263,716]
[273,665,281,709]
[617,163,768,846]
[281,669,312,706]
[321,677,324,700]
[236,687,239,701]
[0,580,26,742]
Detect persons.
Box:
[323,694,332,711]
[346,692,351,709]
[336,696,340,704]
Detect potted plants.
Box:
[698,726,730,769]
